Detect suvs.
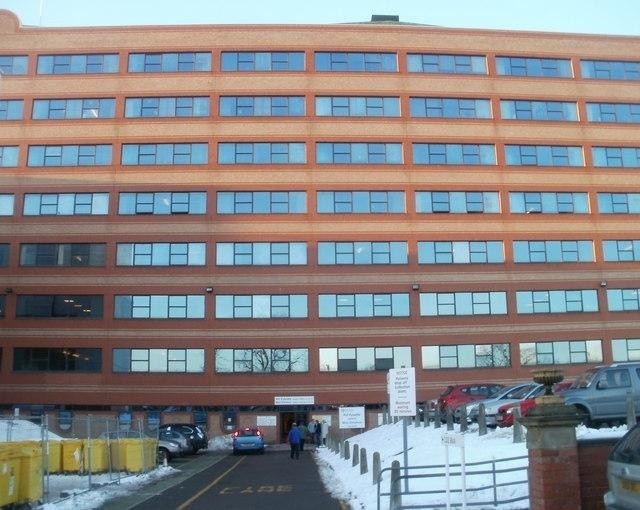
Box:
[93,422,208,463]
[556,361,640,429]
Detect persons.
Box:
[287,422,304,460]
[298,417,329,451]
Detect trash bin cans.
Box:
[0,438,159,510]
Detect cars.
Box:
[232,426,265,454]
[604,417,640,509]
[412,382,590,429]
